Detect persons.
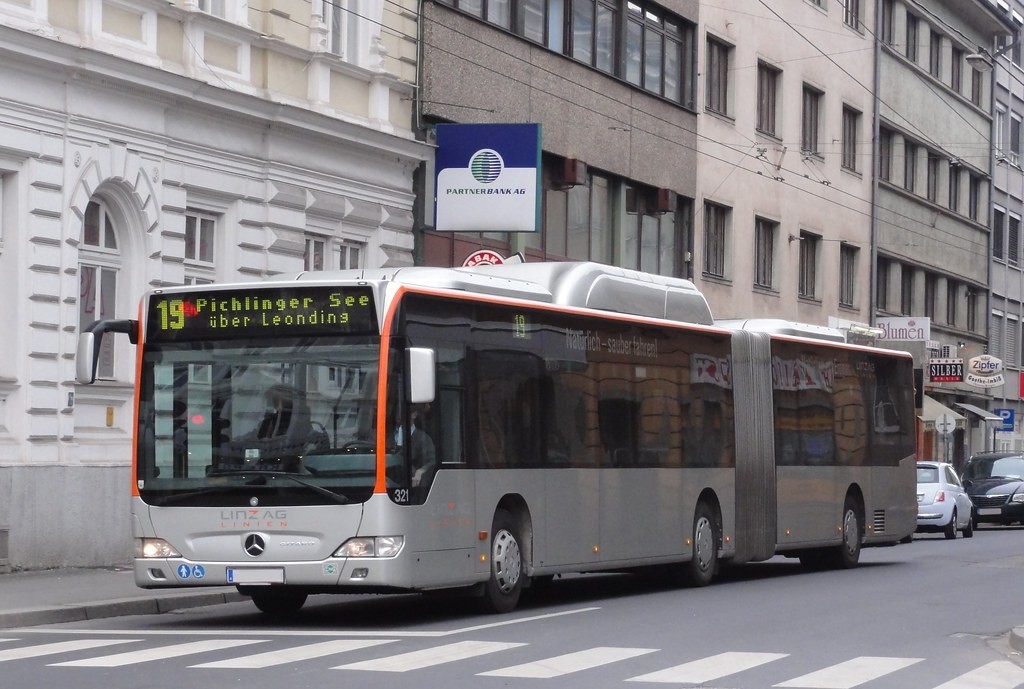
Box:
[387,401,435,487]
[220,384,330,453]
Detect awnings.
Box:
[916,395,966,431]
[954,403,1003,428]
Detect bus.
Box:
[76,258,917,619]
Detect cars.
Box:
[917,460,976,539]
[961,451,1024,528]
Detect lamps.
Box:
[966,46,994,72]
